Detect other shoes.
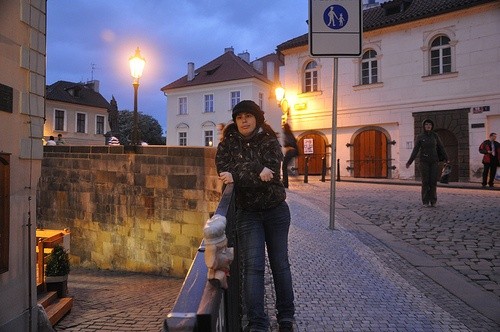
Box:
[423,204,437,207]
[279,321,292,332]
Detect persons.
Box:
[406,118,449,204]
[479,133,500,189]
[42,134,67,147]
[216,100,296,332]
[279,123,298,189]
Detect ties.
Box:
[491,140,495,155]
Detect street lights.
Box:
[128,46,144,145]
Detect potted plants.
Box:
[45,244,71,296]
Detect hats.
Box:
[232,100,264,122]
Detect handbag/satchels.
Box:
[436,144,448,162]
